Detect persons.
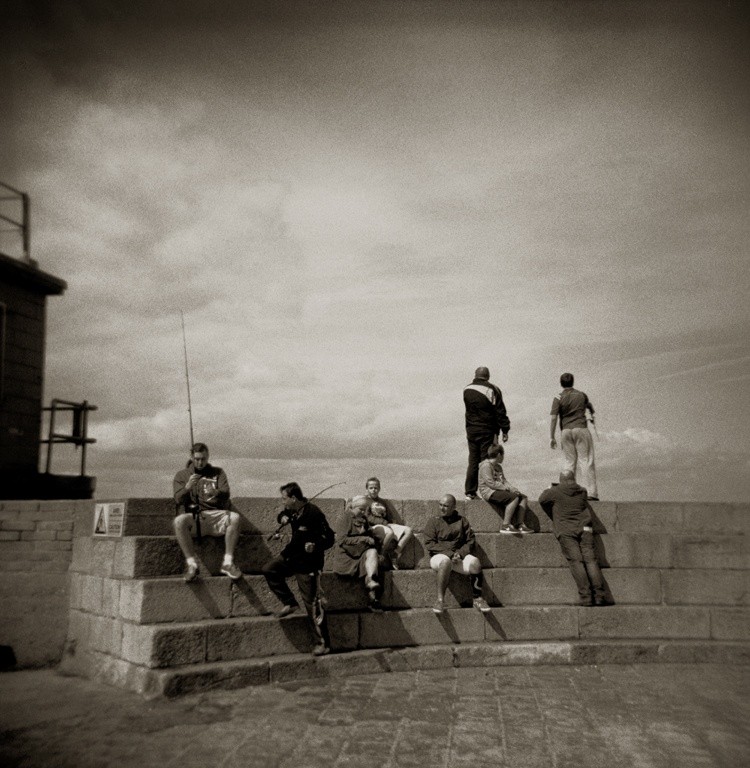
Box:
[539,470,605,606]
[550,372,600,501]
[479,443,534,534]
[463,367,511,500]
[262,483,337,656]
[172,443,242,581]
[332,476,412,613]
[424,494,491,614]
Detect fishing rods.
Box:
[266,481,347,542]
[589,417,601,442]
[179,306,205,548]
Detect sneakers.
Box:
[184,565,200,583]
[220,562,243,579]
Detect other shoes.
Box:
[367,598,383,614]
[465,494,482,501]
[472,598,491,612]
[500,523,521,534]
[388,549,402,569]
[432,599,446,613]
[312,645,331,657]
[515,522,535,534]
[378,554,385,566]
[596,599,616,606]
[278,604,299,618]
[574,599,592,606]
[586,496,599,501]
[365,579,380,588]
